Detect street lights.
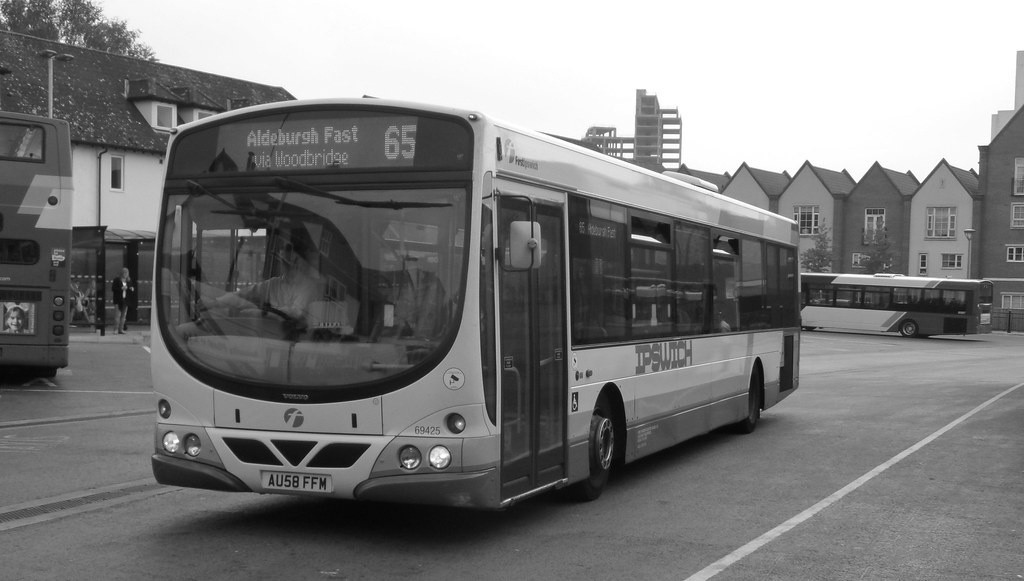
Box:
[964,228,976,278]
[39,48,74,118]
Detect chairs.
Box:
[848,293,960,309]
[570,289,773,339]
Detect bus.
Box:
[801,272,994,337]
[153,97,802,512]
[0,111,74,377]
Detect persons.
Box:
[111,268,134,335]
[2,302,32,334]
[719,308,732,333]
[198,245,320,327]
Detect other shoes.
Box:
[113,329,118,334]
[119,330,126,334]
